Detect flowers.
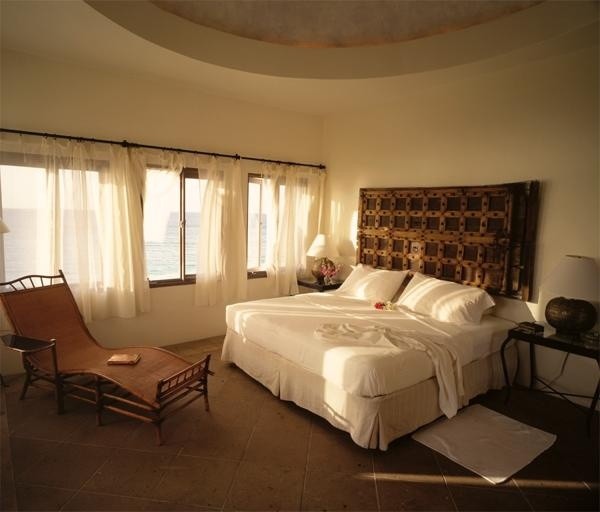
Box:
[319,260,343,278]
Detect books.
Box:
[107,352,141,364]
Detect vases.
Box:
[324,274,331,286]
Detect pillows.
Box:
[336,264,409,303]
[398,272,485,324]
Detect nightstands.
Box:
[298,277,343,292]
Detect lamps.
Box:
[305,233,331,285]
[540,253,600,340]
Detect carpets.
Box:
[413,401,557,488]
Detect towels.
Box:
[315,321,465,418]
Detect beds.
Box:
[224,284,520,448]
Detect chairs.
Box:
[1,268,213,445]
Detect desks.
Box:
[500,320,599,429]
